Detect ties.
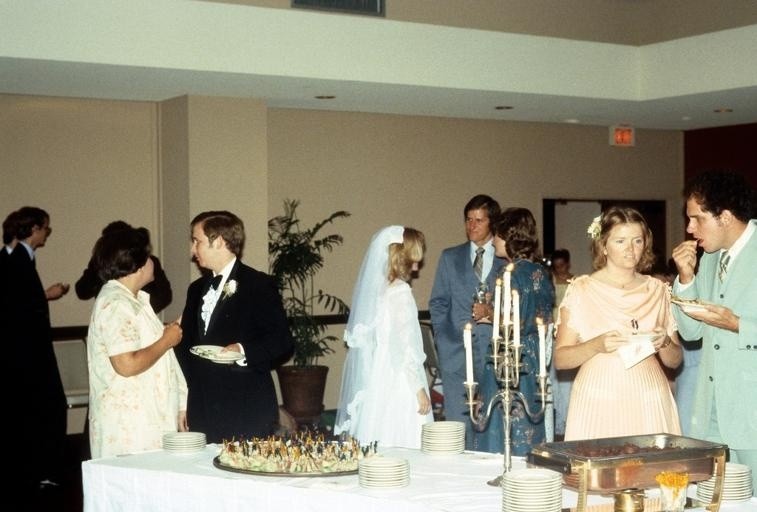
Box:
[472,247,486,281]
[719,250,731,285]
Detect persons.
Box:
[554,210,684,443]
[87,225,191,462]
[473,208,553,453]
[172,211,293,444]
[652,258,678,284]
[3,211,68,299]
[551,249,574,323]
[73,220,172,314]
[1,204,67,499]
[429,196,507,452]
[671,168,755,493]
[345,222,432,456]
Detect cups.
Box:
[659,485,688,512]
[613,487,644,512]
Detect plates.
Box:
[501,468,564,512]
[162,432,207,453]
[420,421,466,455]
[357,456,412,491]
[696,462,755,504]
[189,345,243,365]
[670,299,707,313]
[622,331,664,342]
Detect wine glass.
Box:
[475,283,493,324]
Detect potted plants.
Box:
[268,194,353,430]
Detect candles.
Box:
[536,321,547,378]
[492,279,502,342]
[502,269,513,328]
[511,295,522,347]
[463,318,474,385]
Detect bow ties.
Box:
[206,272,223,291]
